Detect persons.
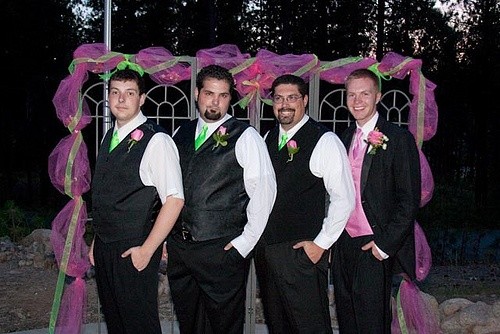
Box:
[161,66,278,334]
[329,68,422,334]
[254,74,357,334]
[90,69,185,334]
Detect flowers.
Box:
[287,140,301,161]
[127,129,144,153]
[367,130,388,154]
[213,126,229,150]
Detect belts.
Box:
[173,226,201,242]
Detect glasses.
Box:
[271,95,304,104]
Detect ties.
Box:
[278,132,288,152]
[353,128,363,160]
[109,130,120,154]
[194,125,208,151]
